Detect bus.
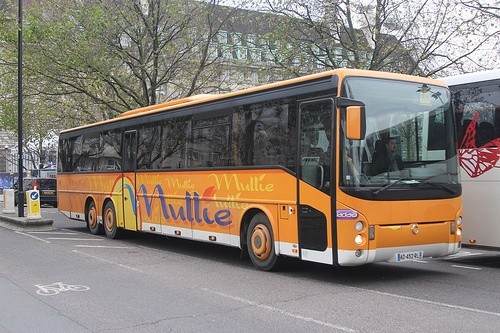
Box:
[432,68,499,248]
[56,68,464,273]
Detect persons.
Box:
[370,137,405,174]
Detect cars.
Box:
[10,178,57,207]
